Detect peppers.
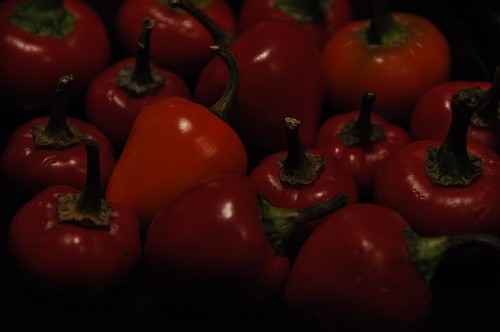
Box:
[0,0,500,332]
